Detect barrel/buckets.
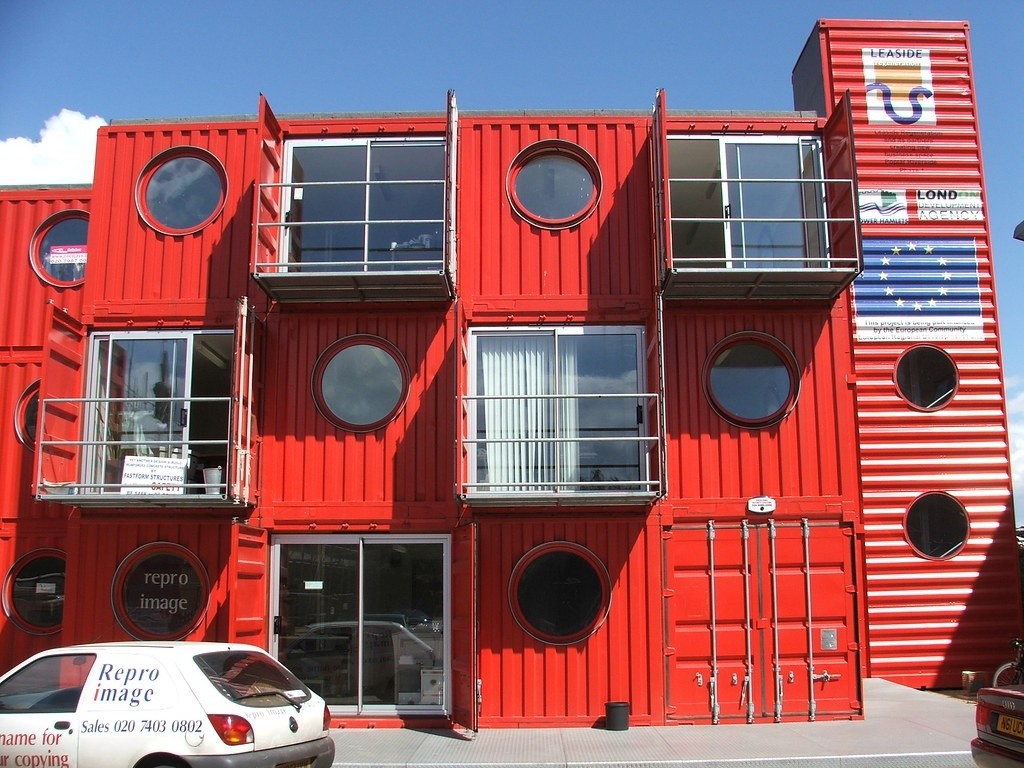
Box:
[202,466,225,495]
[605,703,629,730]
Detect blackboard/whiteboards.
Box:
[120,455,187,495]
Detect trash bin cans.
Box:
[605,701,629,730]
[202,465,223,494]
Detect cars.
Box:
[0,642,335,767]
[278,621,437,703]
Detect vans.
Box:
[355,614,408,636]
